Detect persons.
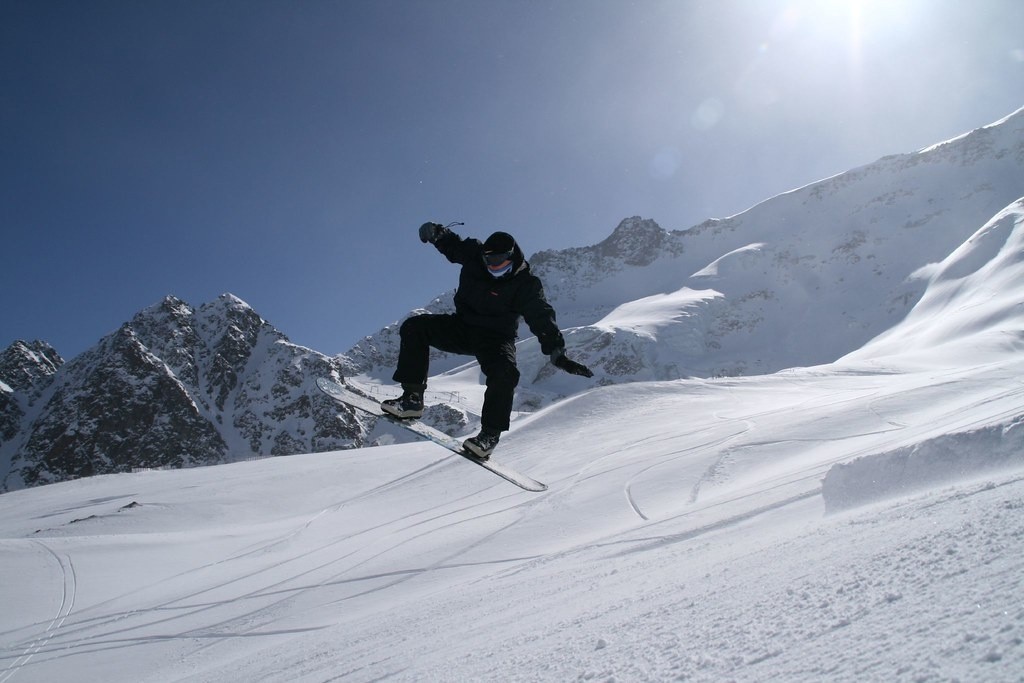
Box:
[380,221,595,459]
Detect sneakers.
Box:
[463,430,500,457]
[381,390,424,417]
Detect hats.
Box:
[482,231,525,274]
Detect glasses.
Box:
[481,251,509,267]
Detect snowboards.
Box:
[315,376,549,492]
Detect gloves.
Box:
[418,222,441,243]
[550,347,594,378]
